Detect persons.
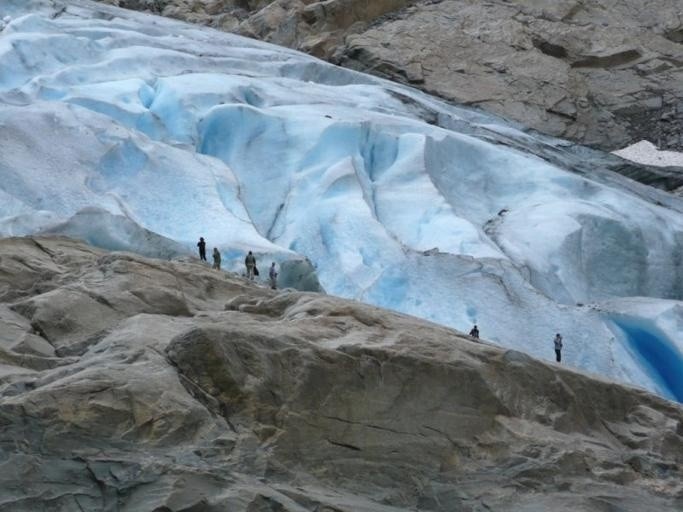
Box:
[468,324,479,339]
[553,332,563,363]
[211,247,221,271]
[268,261,278,289]
[196,237,207,261]
[244,250,256,281]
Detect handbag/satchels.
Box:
[253,266,260,275]
